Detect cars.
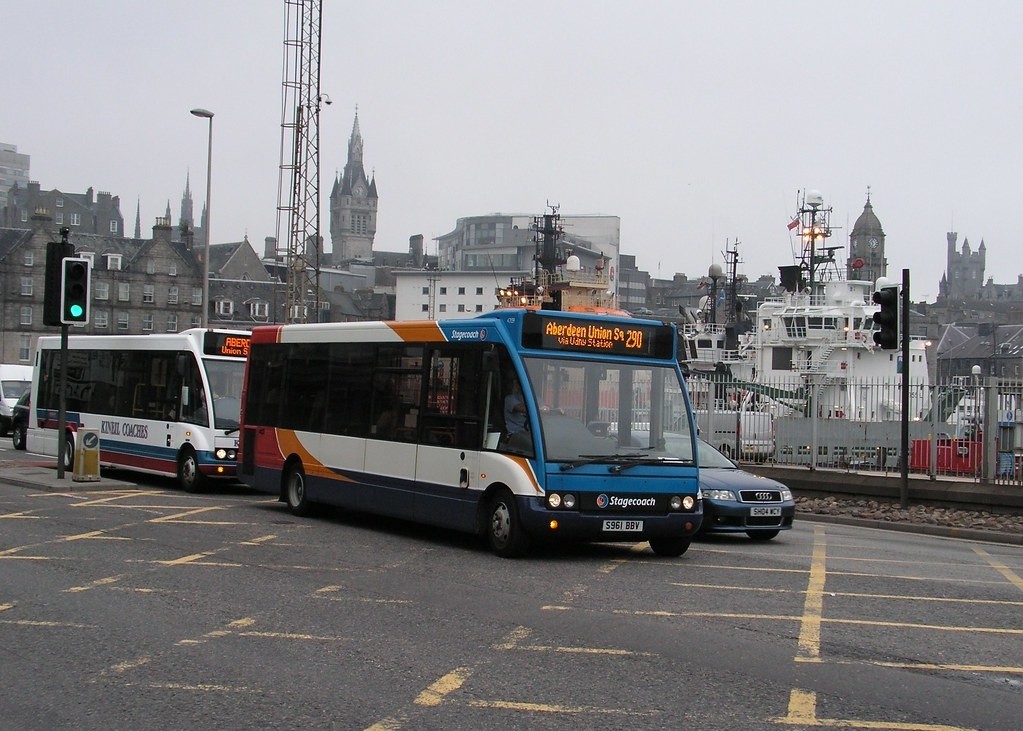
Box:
[11,385,30,450]
[606,430,795,541]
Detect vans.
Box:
[0,364,34,437]
[671,409,776,466]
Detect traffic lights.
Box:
[60,256,91,324]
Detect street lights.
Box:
[190,108,214,327]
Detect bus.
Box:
[24,323,253,493]
[236,307,705,560]
